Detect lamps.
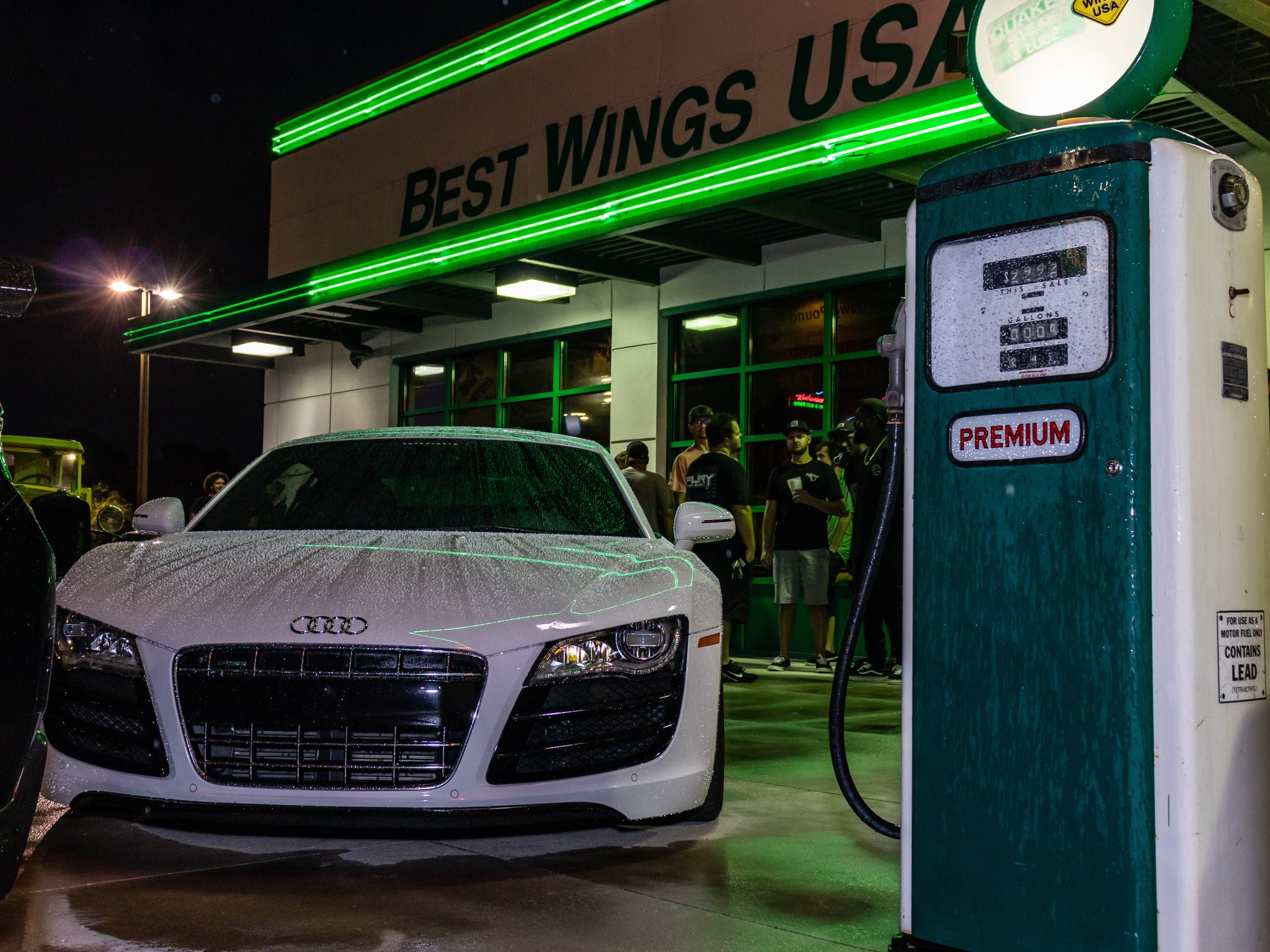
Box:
[495,262,578,302]
[232,331,295,358]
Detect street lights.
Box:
[106,280,185,508]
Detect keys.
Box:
[732,569,735,579]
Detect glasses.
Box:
[692,418,711,426]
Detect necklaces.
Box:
[864,432,887,465]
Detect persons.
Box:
[827,420,856,595]
[668,405,739,509]
[621,440,675,542]
[615,452,627,469]
[805,441,855,666]
[190,471,229,522]
[761,421,847,674]
[844,398,902,684]
[686,413,758,682]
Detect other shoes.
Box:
[805,649,838,666]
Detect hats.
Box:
[859,398,889,426]
[827,421,855,441]
[689,405,713,424]
[622,440,648,459]
[782,420,810,438]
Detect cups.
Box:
[786,476,802,499]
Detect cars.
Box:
[29,422,738,851]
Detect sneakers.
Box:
[722,659,757,682]
[887,664,902,685]
[849,658,888,681]
[815,653,833,673]
[768,656,791,671]
